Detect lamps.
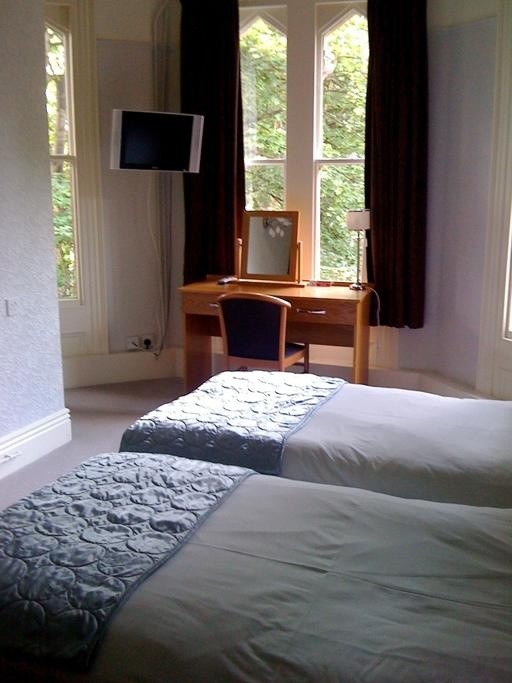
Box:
[347,209,371,291]
[263,216,293,240]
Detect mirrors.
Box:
[234,210,310,288]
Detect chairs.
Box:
[216,290,310,374]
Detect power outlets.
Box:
[141,334,156,350]
[6,297,18,316]
[124,336,140,350]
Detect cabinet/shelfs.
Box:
[178,275,376,396]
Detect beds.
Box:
[0,450,512,683]
[119,370,512,510]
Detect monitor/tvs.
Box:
[109,107,204,174]
